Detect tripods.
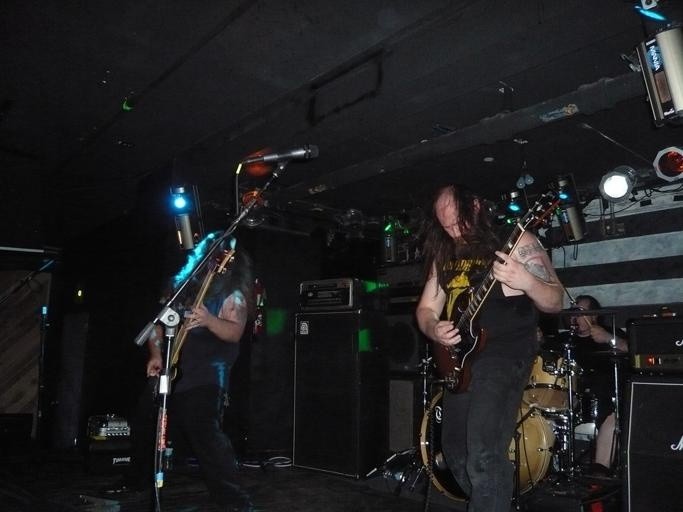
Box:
[551,350,586,483]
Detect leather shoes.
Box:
[99,480,136,494]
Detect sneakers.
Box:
[589,472,607,491]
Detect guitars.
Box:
[434,189,559,394]
[150,249,235,398]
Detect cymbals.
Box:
[559,310,620,313]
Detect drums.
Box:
[573,394,600,441]
[522,356,577,413]
[420,393,555,502]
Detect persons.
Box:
[535,295,630,471]
[414,184,564,512]
[122,252,256,511]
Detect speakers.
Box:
[621,377,682,510]
[292,310,386,481]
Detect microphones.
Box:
[240,142,321,166]
